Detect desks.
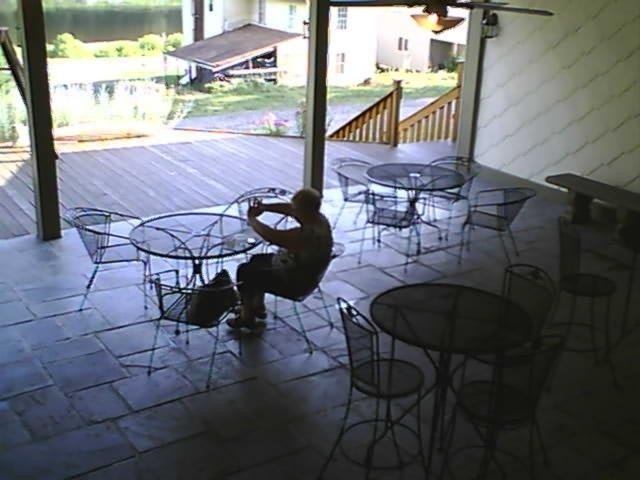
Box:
[129,214,261,340]
[369,282,533,466]
[361,163,466,253]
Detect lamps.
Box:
[480,14,499,41]
[414,5,461,33]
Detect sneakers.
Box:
[227,317,256,329]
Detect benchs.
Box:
[546,171,638,234]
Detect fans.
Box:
[328,0,557,38]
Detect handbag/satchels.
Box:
[190,269,237,322]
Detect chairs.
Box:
[61,208,150,311]
[319,295,427,472]
[143,268,242,389]
[357,189,428,270]
[433,351,561,471]
[408,156,482,242]
[439,263,557,453]
[458,186,537,268]
[546,214,626,393]
[241,243,356,353]
[329,157,402,237]
[205,184,297,279]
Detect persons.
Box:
[225,185,336,330]
